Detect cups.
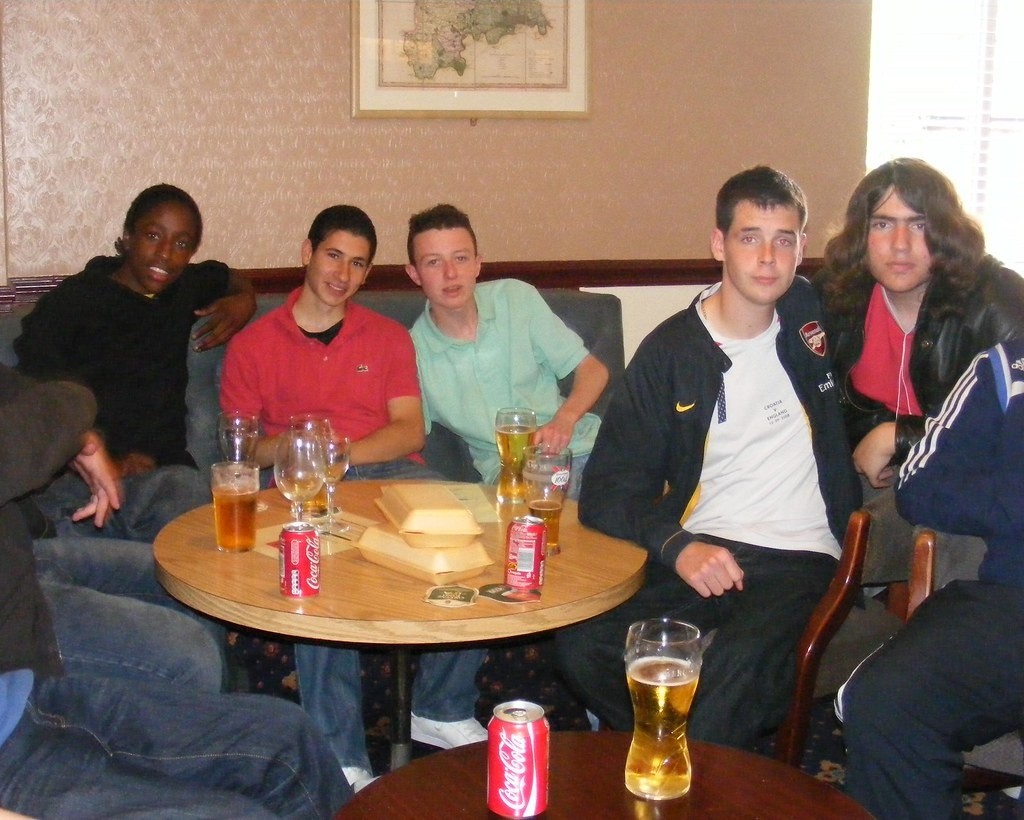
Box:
[624,617,704,802]
[522,444,573,557]
[288,414,333,519]
[210,462,260,553]
[497,406,538,507]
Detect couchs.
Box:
[183,287,625,501]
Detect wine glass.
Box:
[216,409,268,513]
[310,430,351,534]
[273,429,325,538]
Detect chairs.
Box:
[777,464,911,766]
[910,522,1024,779]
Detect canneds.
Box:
[487,700,550,819]
[504,516,548,590]
[278,522,321,598]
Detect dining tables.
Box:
[150,480,647,770]
[330,734,875,820]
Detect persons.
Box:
[220,205,489,794]
[405,204,609,731]
[551,164,863,751]
[12,183,257,544]
[0,361,356,820]
[808,158,1024,488]
[834,338,1024,819]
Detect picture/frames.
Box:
[349,0,592,120]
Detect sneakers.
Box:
[411,709,489,749]
[341,765,385,794]
